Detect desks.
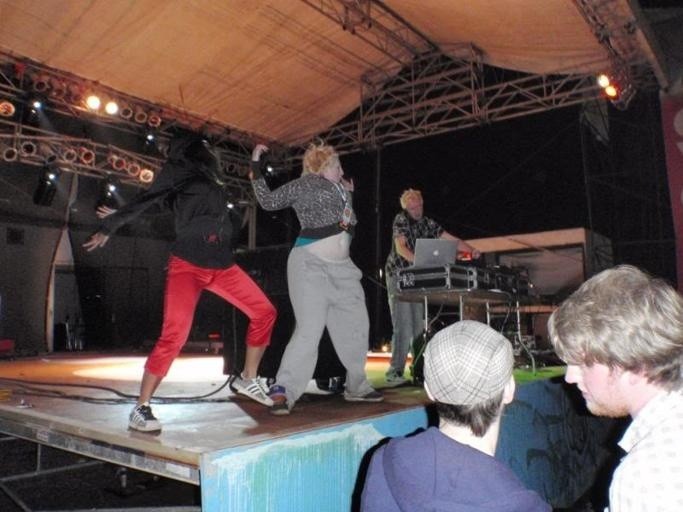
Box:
[396,286,537,381]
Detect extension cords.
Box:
[489,288,502,294]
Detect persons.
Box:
[249,140,385,417]
[81,133,278,432]
[546,264,683,512]
[385,187,481,384]
[361,319,553,512]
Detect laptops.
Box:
[400,239,458,269]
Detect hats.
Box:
[423,318,515,405]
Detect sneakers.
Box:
[267,385,290,415]
[128,403,160,433]
[386,368,412,389]
[345,388,384,402]
[231,372,275,407]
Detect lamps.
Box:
[75,143,94,166]
[117,99,132,120]
[50,79,68,100]
[100,92,120,116]
[29,72,49,93]
[124,155,141,176]
[602,79,627,96]
[106,152,125,172]
[609,85,637,111]
[94,177,119,214]
[145,107,163,129]
[40,138,58,164]
[598,68,620,87]
[80,86,103,111]
[33,170,59,207]
[17,137,36,158]
[136,163,154,184]
[53,137,78,162]
[0,141,17,163]
[132,102,148,124]
